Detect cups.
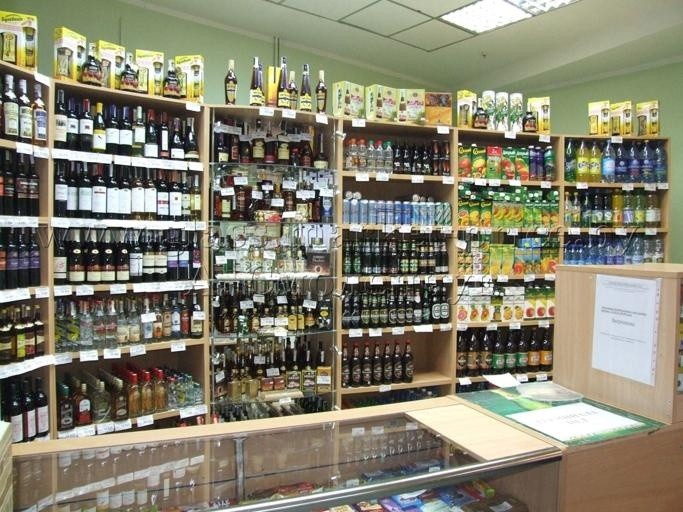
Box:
[459,105,466,126]
[638,116,645,134]
[1,32,16,63]
[587,115,597,135]
[612,116,620,136]
[55,54,68,77]
[177,73,186,97]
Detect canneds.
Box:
[342,189,452,226]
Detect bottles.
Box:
[473,98,487,129]
[224,59,236,105]
[11,422,448,511]
[542,103,550,132]
[522,101,537,132]
[601,109,607,134]
[24,26,35,68]
[338,127,456,405]
[0,74,49,447]
[81,43,181,100]
[650,107,657,136]
[55,88,207,441]
[75,37,85,81]
[249,56,327,115]
[624,109,630,134]
[192,65,200,98]
[564,190,666,263]
[210,115,342,425]
[528,141,667,187]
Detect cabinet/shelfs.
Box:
[450,259,683,512]
[0,62,669,456]
[2,394,562,511]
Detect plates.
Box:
[514,382,583,404]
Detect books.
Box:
[515,378,584,405]
